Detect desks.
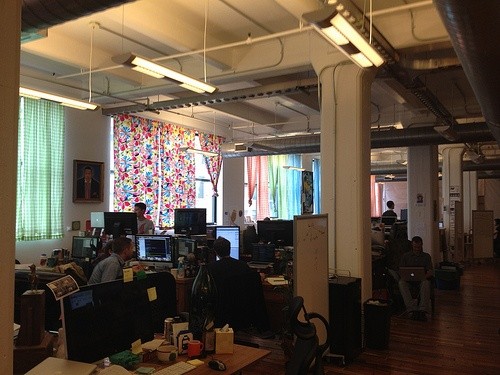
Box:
[14,247,293,375]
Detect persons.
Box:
[89,237,141,284]
[87,241,112,280]
[399,236,434,321]
[493,219,500,258]
[208,238,264,297]
[382,201,397,218]
[133,202,155,234]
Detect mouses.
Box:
[209,359,226,371]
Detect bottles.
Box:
[187,264,217,339]
[40,253,48,269]
[201,303,215,355]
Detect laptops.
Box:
[398,266,425,283]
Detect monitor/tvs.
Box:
[71,236,98,262]
[215,226,240,261]
[257,220,293,249]
[60,270,178,364]
[104,211,138,238]
[175,208,206,238]
[134,235,172,262]
[176,239,196,256]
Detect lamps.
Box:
[282,161,305,172]
[301,0,387,69]
[468,143,486,166]
[111,0,218,95]
[178,146,218,159]
[19,26,101,111]
[433,115,460,141]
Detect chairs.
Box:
[58,261,93,286]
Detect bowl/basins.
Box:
[157,345,178,364]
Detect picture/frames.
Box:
[73,159,105,203]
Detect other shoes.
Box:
[418,311,426,321]
[409,312,418,320]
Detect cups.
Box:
[47,257,56,267]
[170,269,179,279]
[187,340,204,358]
